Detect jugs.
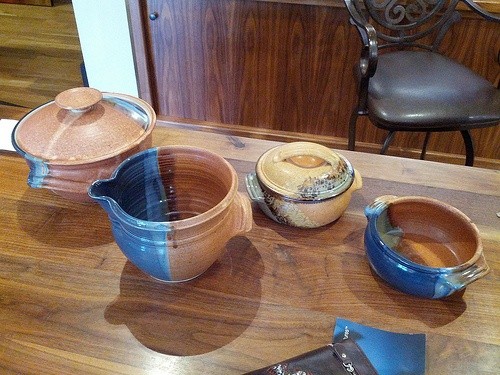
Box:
[88,145,252,283]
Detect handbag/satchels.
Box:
[241,337,378,375]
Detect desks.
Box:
[0,105,500,375]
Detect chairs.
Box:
[344,0,500,166]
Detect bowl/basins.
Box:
[363,194,490,300]
[244,142,363,229]
[12,87,156,204]
